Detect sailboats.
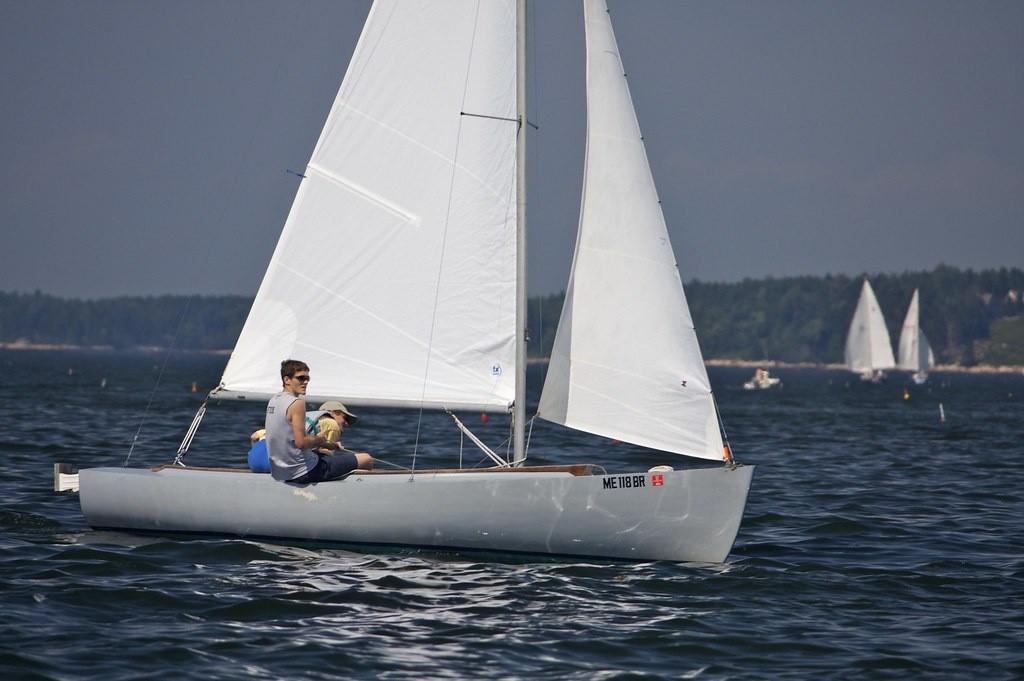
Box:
[893,286,944,403]
[837,277,896,402]
[55,0,757,571]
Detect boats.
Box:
[744,378,783,389]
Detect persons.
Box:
[265,360,373,485]
[246,402,358,473]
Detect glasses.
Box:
[291,375,310,382]
[339,413,350,424]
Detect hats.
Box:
[319,402,358,425]
